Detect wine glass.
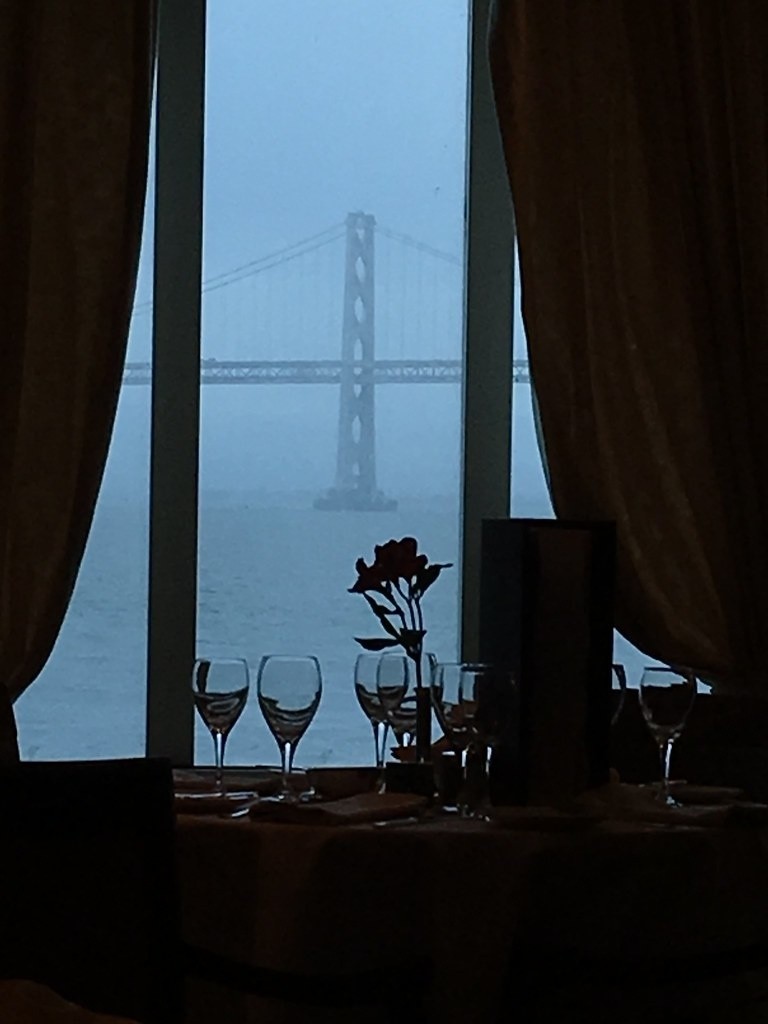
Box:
[258,656,322,801]
[354,654,408,793]
[636,666,698,811]
[575,664,627,791]
[374,653,441,795]
[431,663,485,812]
[191,659,250,802]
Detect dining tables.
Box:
[178,795,768,1000]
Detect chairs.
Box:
[1,757,188,1019]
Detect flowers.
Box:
[348,539,455,688]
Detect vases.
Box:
[413,687,431,762]
